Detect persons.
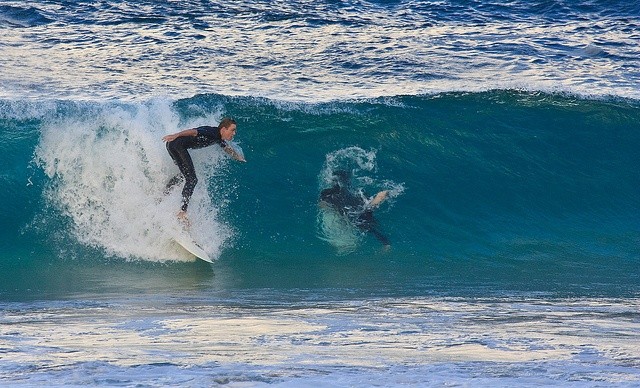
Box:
[155,118,247,225]
[321,170,392,253]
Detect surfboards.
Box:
[154,197,215,264]
